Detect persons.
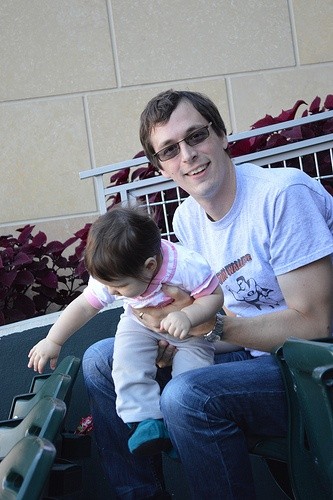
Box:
[27,210,223,453]
[83,88,332,499]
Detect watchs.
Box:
[203,310,223,343]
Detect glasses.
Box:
[152,121,213,162]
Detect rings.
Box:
[139,312,143,319]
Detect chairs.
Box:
[0,336,333,500]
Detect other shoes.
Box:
[128,419,170,457]
